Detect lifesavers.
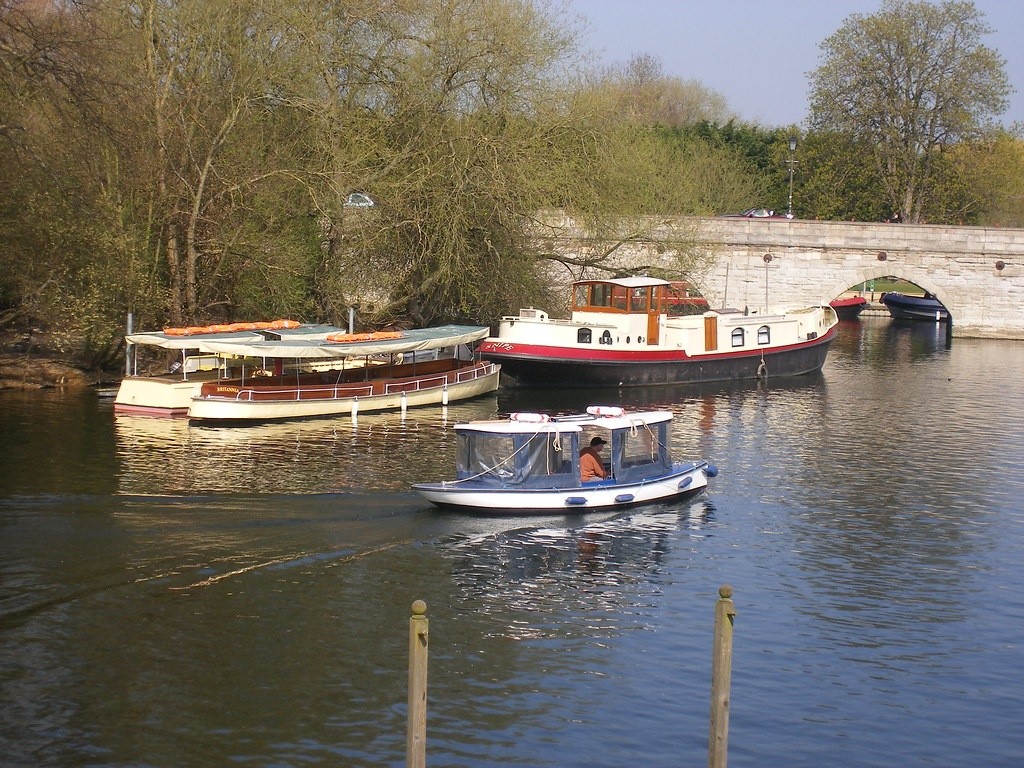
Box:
[510,413,552,423]
[163,319,303,336]
[585,406,625,417]
[327,332,401,340]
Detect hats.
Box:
[590,437,607,446]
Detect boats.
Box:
[409,406,719,518]
[474,277,841,391]
[112,319,502,427]
[828,295,866,321]
[94,387,119,397]
[878,291,949,322]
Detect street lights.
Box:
[787,132,799,219]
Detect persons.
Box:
[580,437,608,483]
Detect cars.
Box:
[343,192,375,208]
[721,206,789,219]
